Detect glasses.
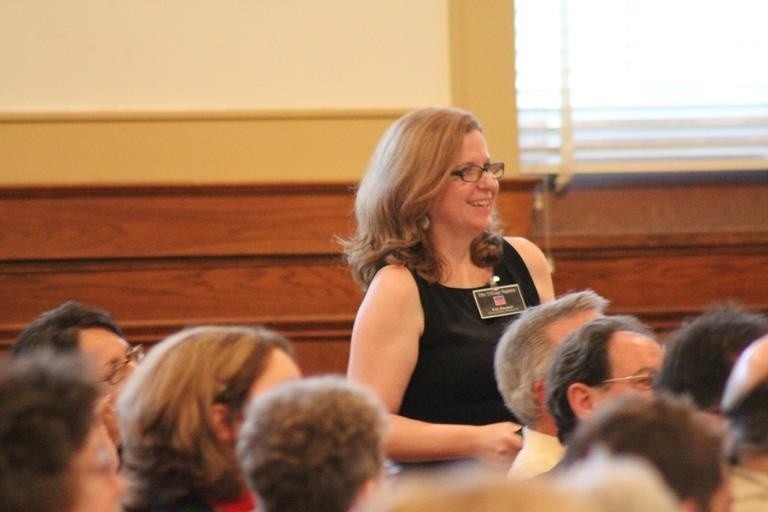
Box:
[99,343,146,385]
[451,161,505,183]
[603,373,653,393]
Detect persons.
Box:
[563,441,680,511]
[352,466,600,511]
[330,105,556,484]
[493,288,767,511]
[1,299,390,511]
